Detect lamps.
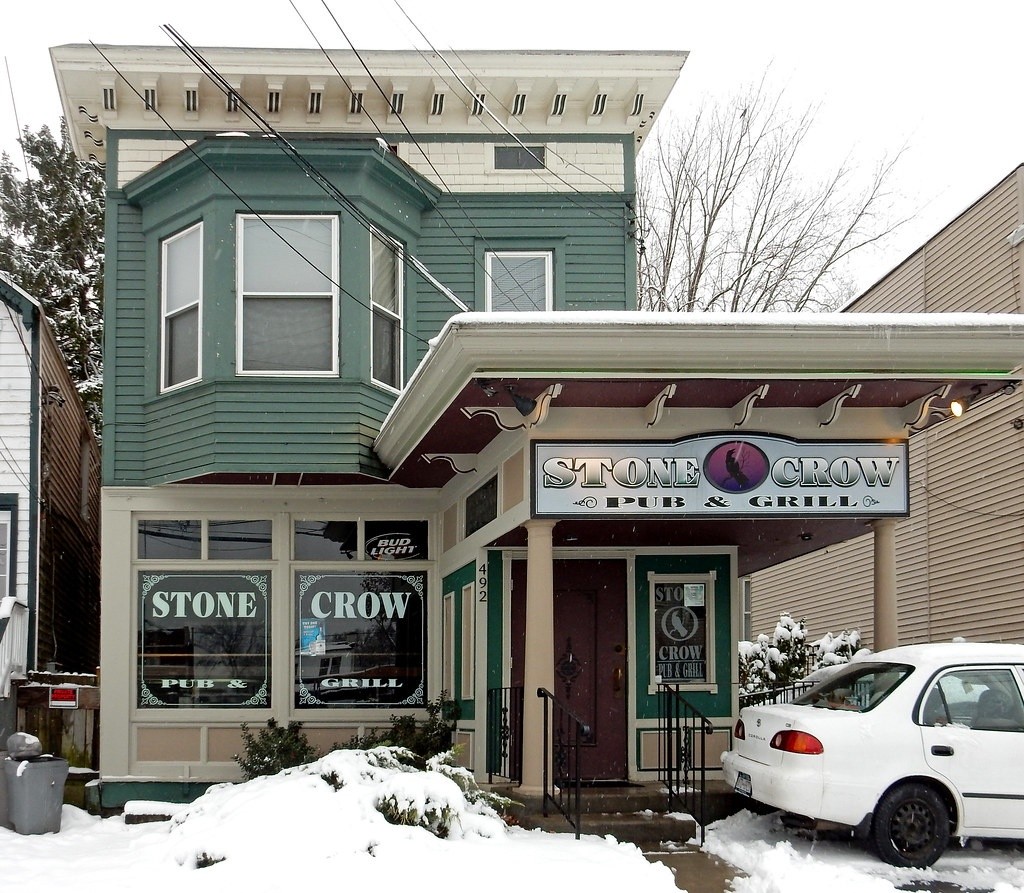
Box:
[504,383,538,417]
[951,382,989,416]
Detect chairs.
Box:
[972,689,1021,729]
[922,688,948,727]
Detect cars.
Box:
[719,643,1024,866]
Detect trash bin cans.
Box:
[0,751,11,826]
[3,757,69,835]
